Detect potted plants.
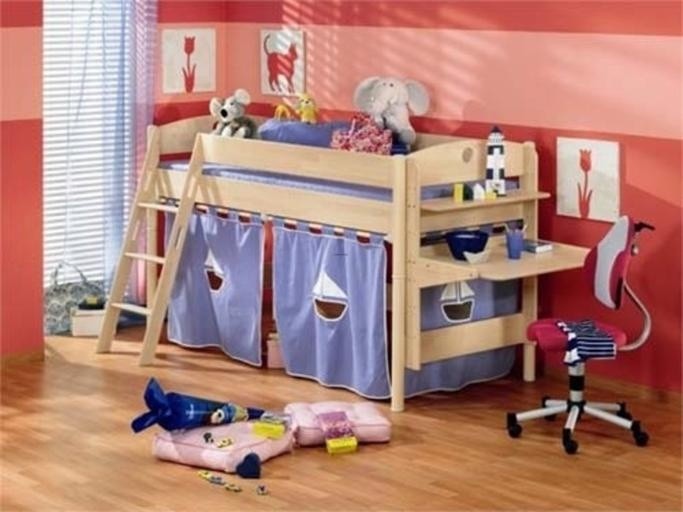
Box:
[68,296,118,337]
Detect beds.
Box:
[95,114,537,413]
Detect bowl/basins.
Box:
[445,230,489,261]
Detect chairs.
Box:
[504,215,655,455]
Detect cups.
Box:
[504,230,524,260]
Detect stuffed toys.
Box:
[353,76,430,153]
[296,94,318,124]
[205,88,255,138]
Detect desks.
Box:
[397,233,592,413]
